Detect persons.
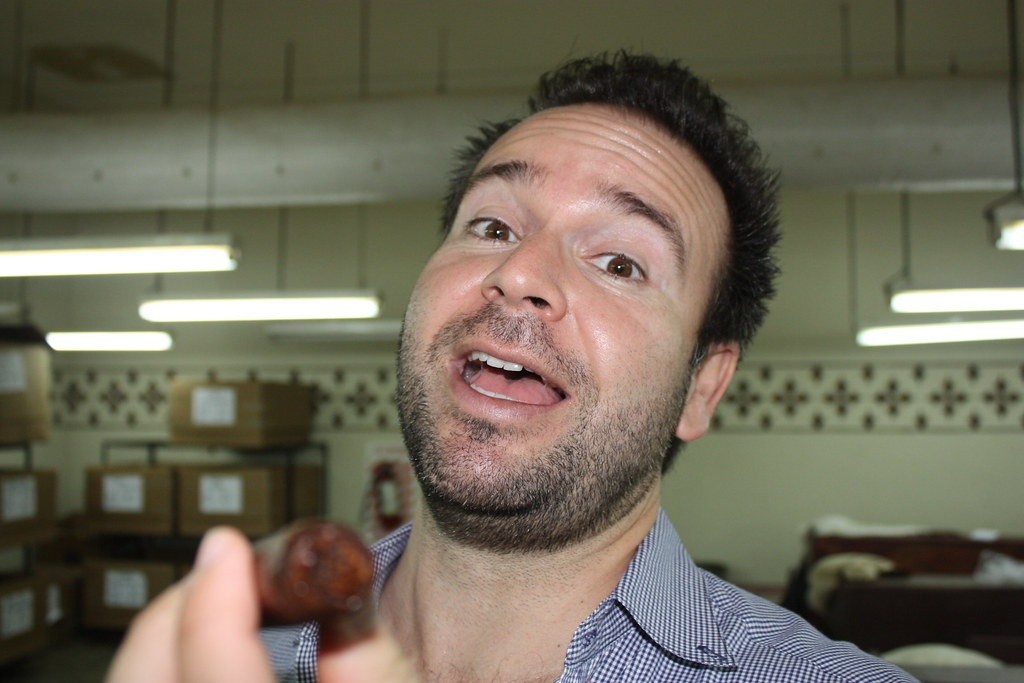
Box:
[106,47,918,682]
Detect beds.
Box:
[801,512,1024,668]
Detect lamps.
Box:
[0,231,240,278]
[883,277,1023,312]
[990,197,1024,251]
[138,288,381,322]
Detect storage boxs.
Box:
[0,324,325,666]
